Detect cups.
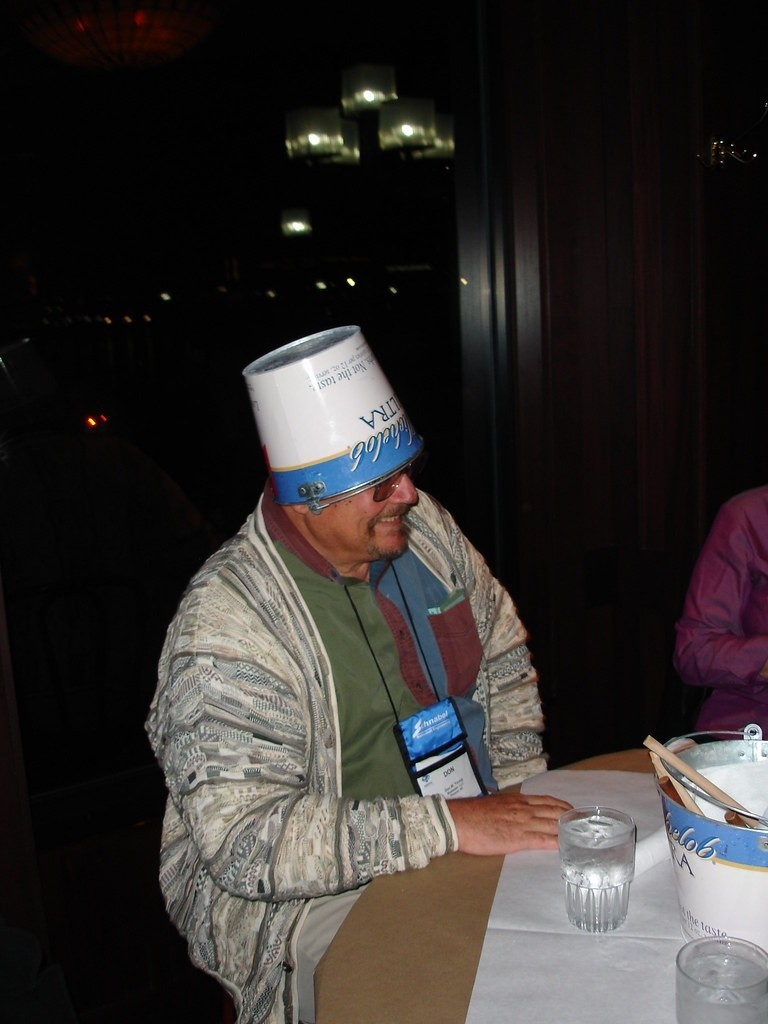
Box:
[674,938,768,1024]
[558,807,636,933]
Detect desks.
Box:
[313,750,768,1024]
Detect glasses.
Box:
[366,463,413,502]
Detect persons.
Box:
[142,325,587,1024]
[672,486,768,744]
[0,339,219,735]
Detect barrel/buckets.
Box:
[651,729,768,946]
[246,332,421,514]
